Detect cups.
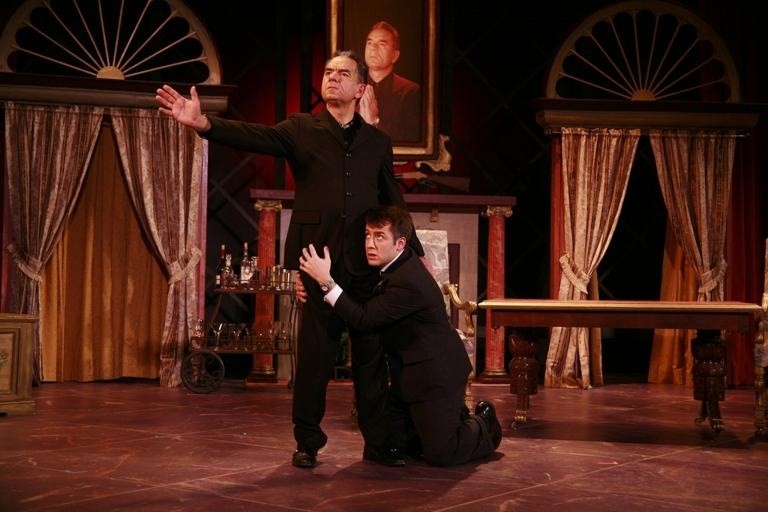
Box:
[265,265,291,289]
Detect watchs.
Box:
[320,278,334,293]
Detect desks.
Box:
[478,299,762,441]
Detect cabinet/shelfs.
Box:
[0,313,40,416]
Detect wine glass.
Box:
[191,319,289,352]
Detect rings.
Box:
[302,261,306,267]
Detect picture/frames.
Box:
[326,0,440,160]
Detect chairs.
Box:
[436,283,479,416]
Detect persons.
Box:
[355,21,421,148]
[154,50,436,468]
[297,205,503,467]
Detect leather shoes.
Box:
[475,399,502,451]
[363,449,406,467]
[293,445,319,469]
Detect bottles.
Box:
[215,242,263,290]
